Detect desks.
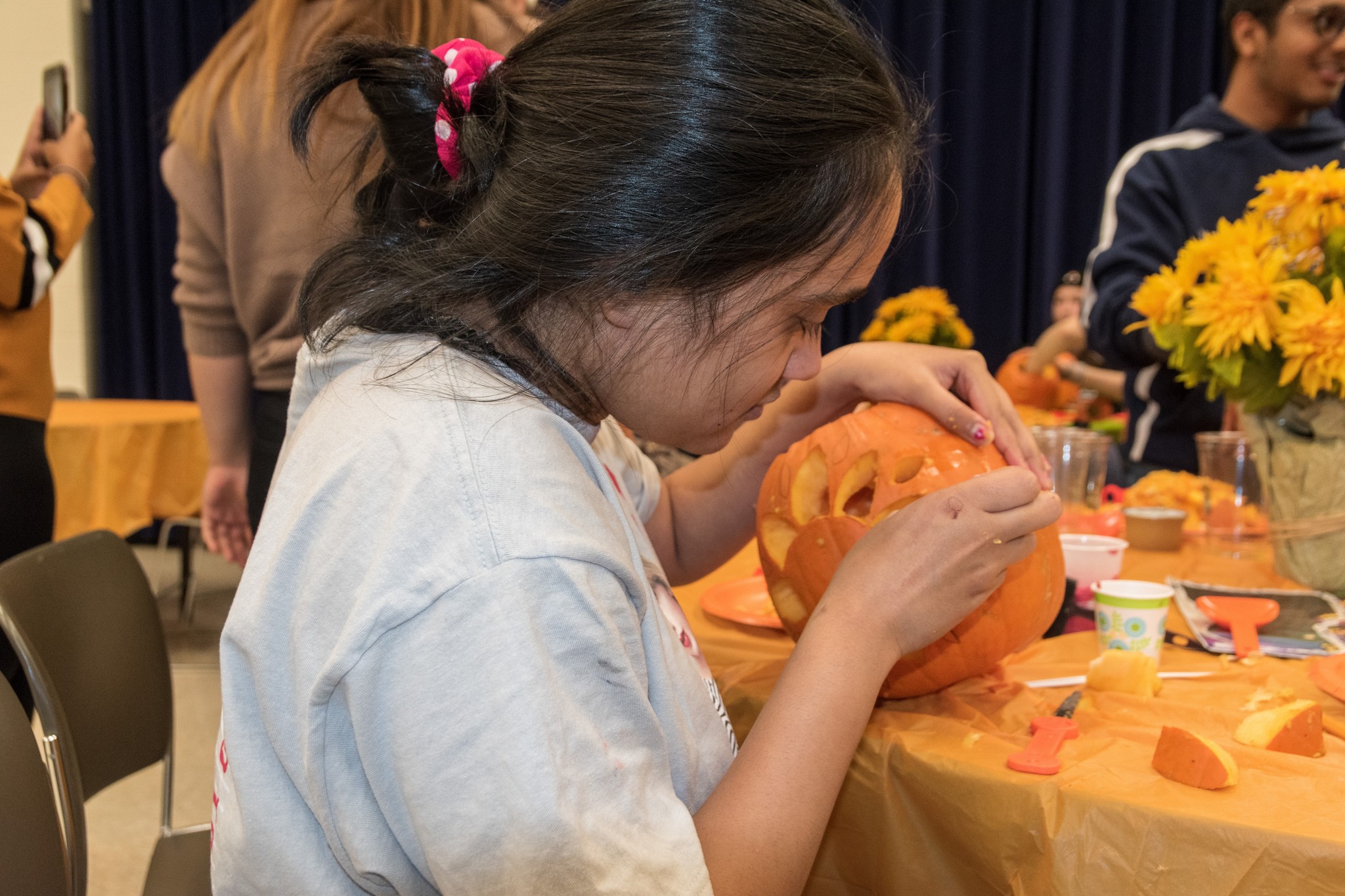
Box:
[672,490,1345,896]
[43,399,211,623]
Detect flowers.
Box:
[857,285,976,349]
[1122,155,1345,560]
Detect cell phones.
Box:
[41,63,67,167]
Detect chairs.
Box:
[0,526,213,896]
[0,668,72,896]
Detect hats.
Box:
[1054,270,1084,287]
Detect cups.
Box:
[1028,423,1114,515]
[1090,579,1175,665]
[1059,532,1130,634]
[1124,506,1187,553]
[1198,429,1266,541]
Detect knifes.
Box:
[1006,690,1082,775]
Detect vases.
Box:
[1231,398,1345,599]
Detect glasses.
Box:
[1313,3,1345,41]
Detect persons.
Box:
[162,0,553,570]
[0,93,96,725]
[1081,0,1344,486]
[1026,261,1225,490]
[207,1,1062,896]
